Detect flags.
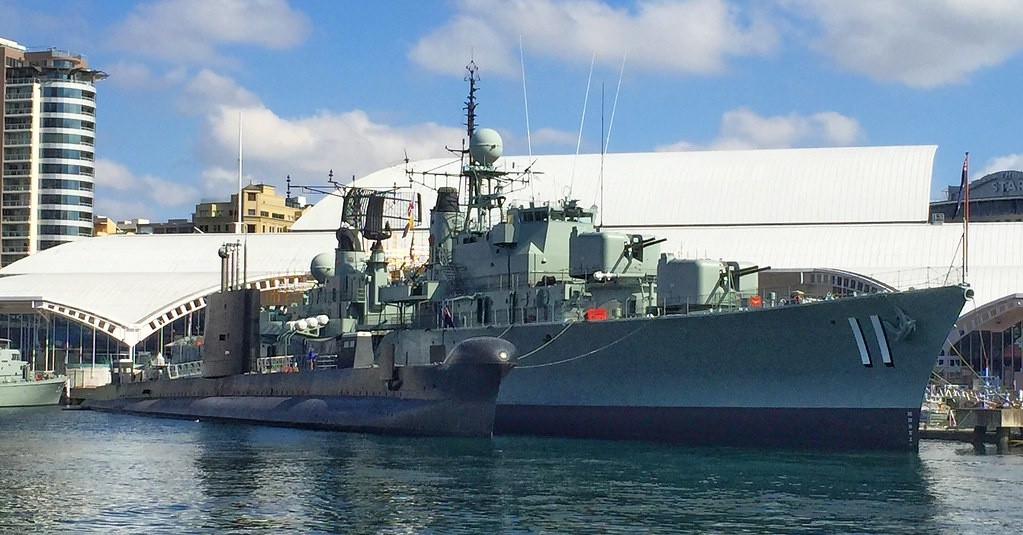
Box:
[408,194,415,216]
[402,214,414,238]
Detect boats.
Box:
[0,337,66,407]
[266,32,973,454]
[88,226,519,439]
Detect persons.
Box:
[443,307,454,328]
[307,347,316,369]
